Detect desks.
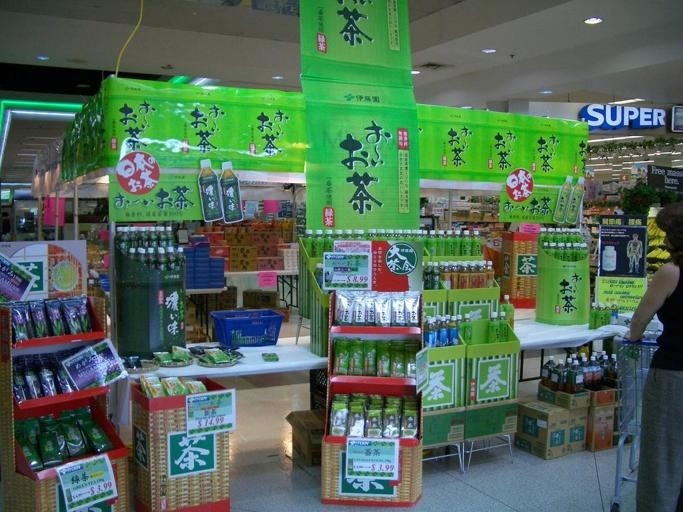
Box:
[223,269,300,308]
[186,287,228,340]
[110,344,329,429]
[512,309,663,351]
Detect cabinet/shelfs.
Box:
[419,195,512,231]
[295,238,520,473]
[0,291,133,512]
[320,291,424,507]
[583,182,672,301]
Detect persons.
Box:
[624,204,683,512]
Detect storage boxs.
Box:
[200,218,300,271]
[513,381,616,460]
[285,408,327,469]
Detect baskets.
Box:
[210,308,285,348]
[500,232,537,309]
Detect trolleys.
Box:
[607,327,662,512]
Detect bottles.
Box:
[541,351,617,394]
[314,263,321,281]
[539,227,589,262]
[87,268,100,296]
[552,176,573,224]
[589,303,618,329]
[302,230,481,257]
[219,162,243,222]
[317,264,323,288]
[197,159,223,222]
[114,227,184,271]
[566,177,586,223]
[602,246,617,271]
[424,295,514,347]
[422,260,495,289]
